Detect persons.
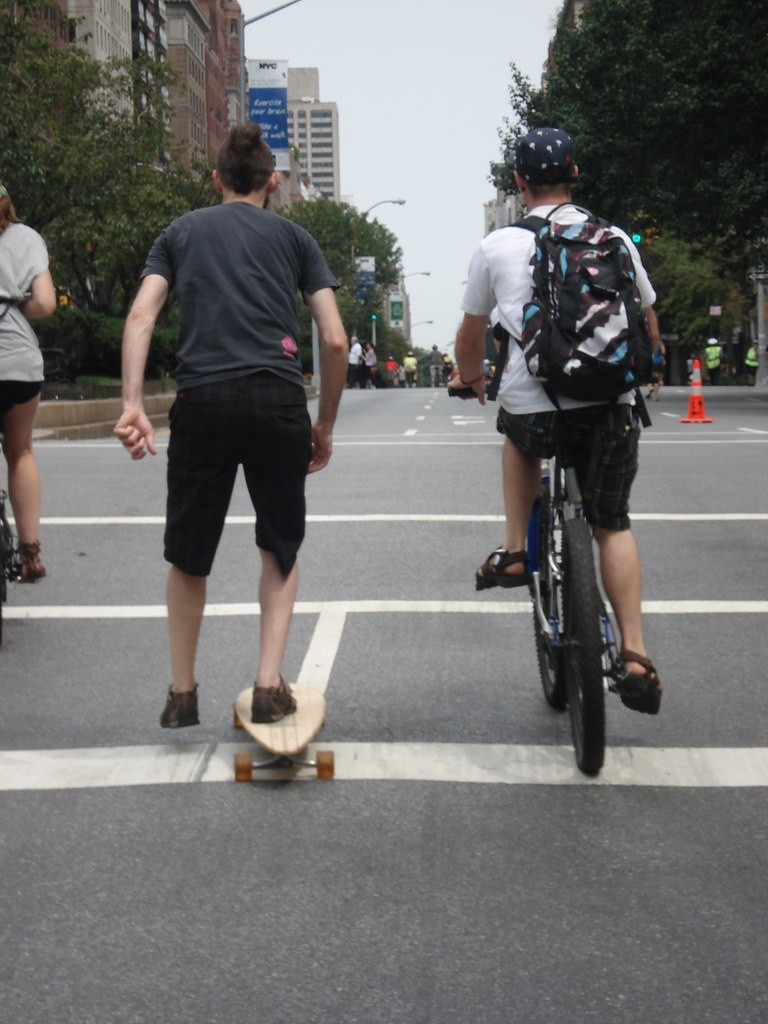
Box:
[0,183,57,581]
[443,354,453,377]
[744,340,759,385]
[449,128,663,714]
[704,338,723,385]
[114,123,348,728]
[429,344,444,386]
[686,354,694,374]
[385,357,399,386]
[346,336,378,389]
[646,340,666,401]
[483,361,496,378]
[398,363,405,388]
[404,352,417,388]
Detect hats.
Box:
[407,350,414,356]
[708,338,718,345]
[507,126,575,185]
[753,339,758,344]
[0,183,8,198]
[388,356,394,360]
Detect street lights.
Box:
[385,272,431,330]
[349,197,408,277]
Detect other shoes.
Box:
[18,541,46,580]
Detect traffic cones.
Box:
[679,358,714,424]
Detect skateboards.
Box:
[233,682,334,781]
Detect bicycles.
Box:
[432,366,441,388]
[443,369,668,777]
[0,436,44,650]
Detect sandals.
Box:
[475,548,530,590]
[616,648,661,715]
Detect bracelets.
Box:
[460,377,481,385]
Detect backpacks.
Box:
[499,203,656,403]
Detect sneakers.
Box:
[251,672,297,723]
[159,681,201,729]
